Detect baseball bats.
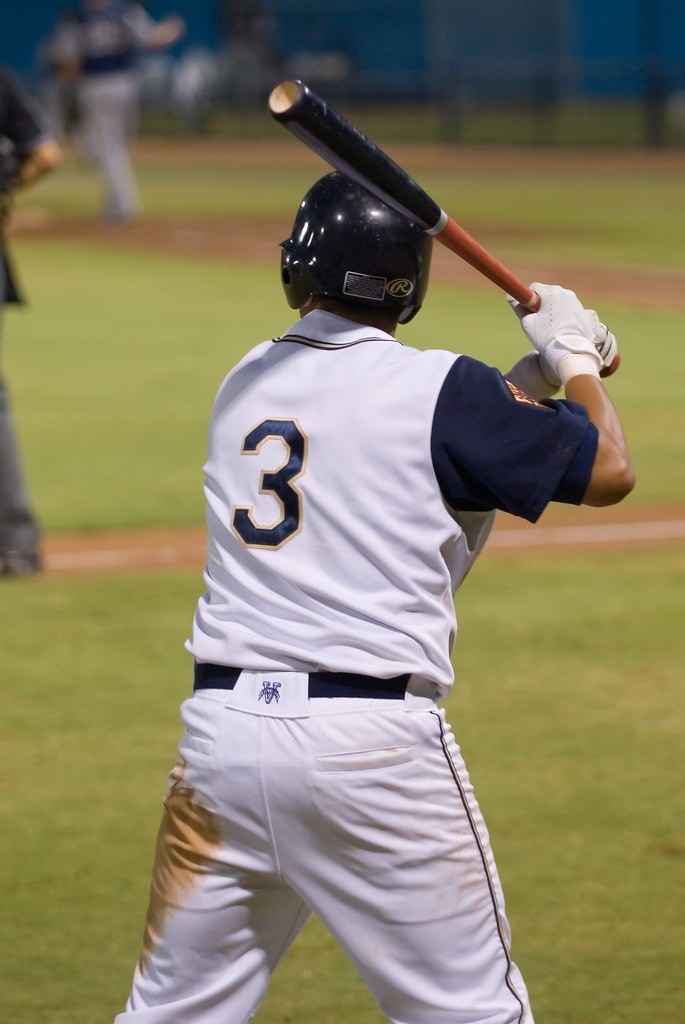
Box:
[264,76,626,378]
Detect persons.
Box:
[0,0,186,578]
[112,173,636,1024]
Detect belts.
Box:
[193,661,412,701]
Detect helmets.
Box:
[280,170,432,325]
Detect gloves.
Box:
[506,282,603,385]
[538,307,618,387]
